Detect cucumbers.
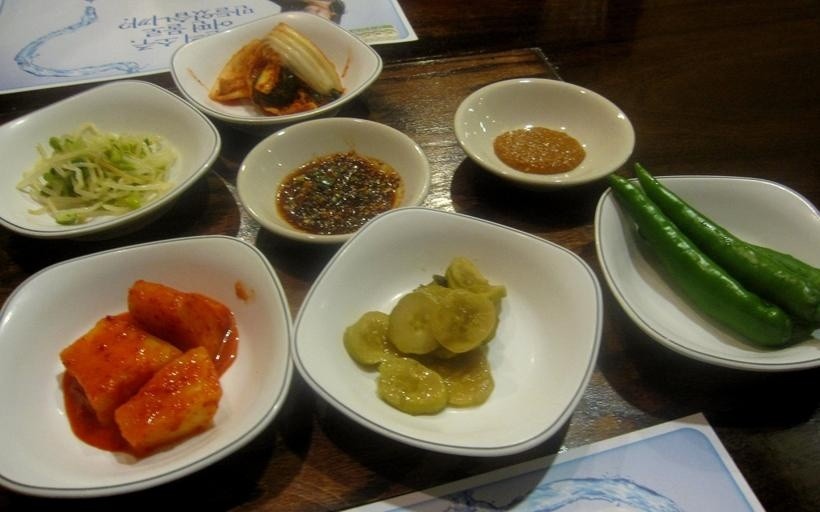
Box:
[342,258,509,418]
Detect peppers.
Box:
[604,159,815,349]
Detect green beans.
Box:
[16,121,175,227]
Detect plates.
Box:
[595,176,820,374]
[236,116,429,249]
[294,208,604,457]
[454,78,636,185]
[170,11,385,124]
[1,79,221,239]
[1,235,293,497]
